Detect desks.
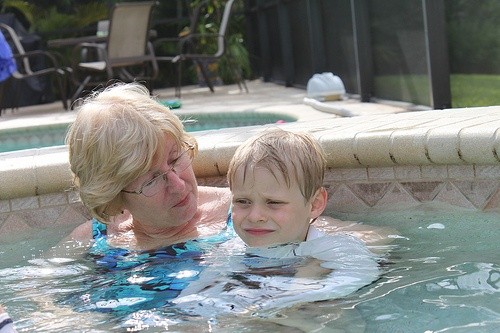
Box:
[48,29,158,95]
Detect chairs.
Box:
[69,0,161,108]
[0,16,73,115]
[150,2,214,97]
[172,0,251,98]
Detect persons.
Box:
[39,84,399,333]
[134,127,382,320]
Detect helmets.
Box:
[306,71,346,101]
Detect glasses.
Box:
[122,140,198,198]
